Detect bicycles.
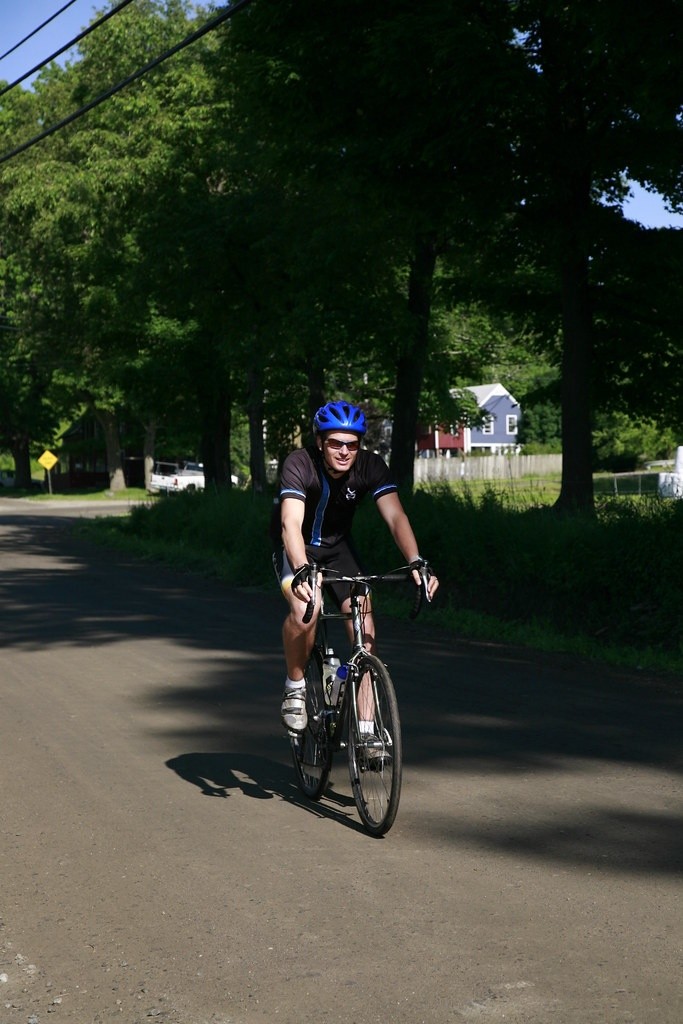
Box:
[287,557,434,837]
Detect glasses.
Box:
[321,436,359,450]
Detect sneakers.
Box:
[357,732,392,766]
[281,674,310,734]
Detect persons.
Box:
[269,401,439,769]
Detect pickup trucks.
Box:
[150,460,242,494]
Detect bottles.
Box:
[329,664,348,707]
[322,648,341,706]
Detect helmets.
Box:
[313,400,366,436]
